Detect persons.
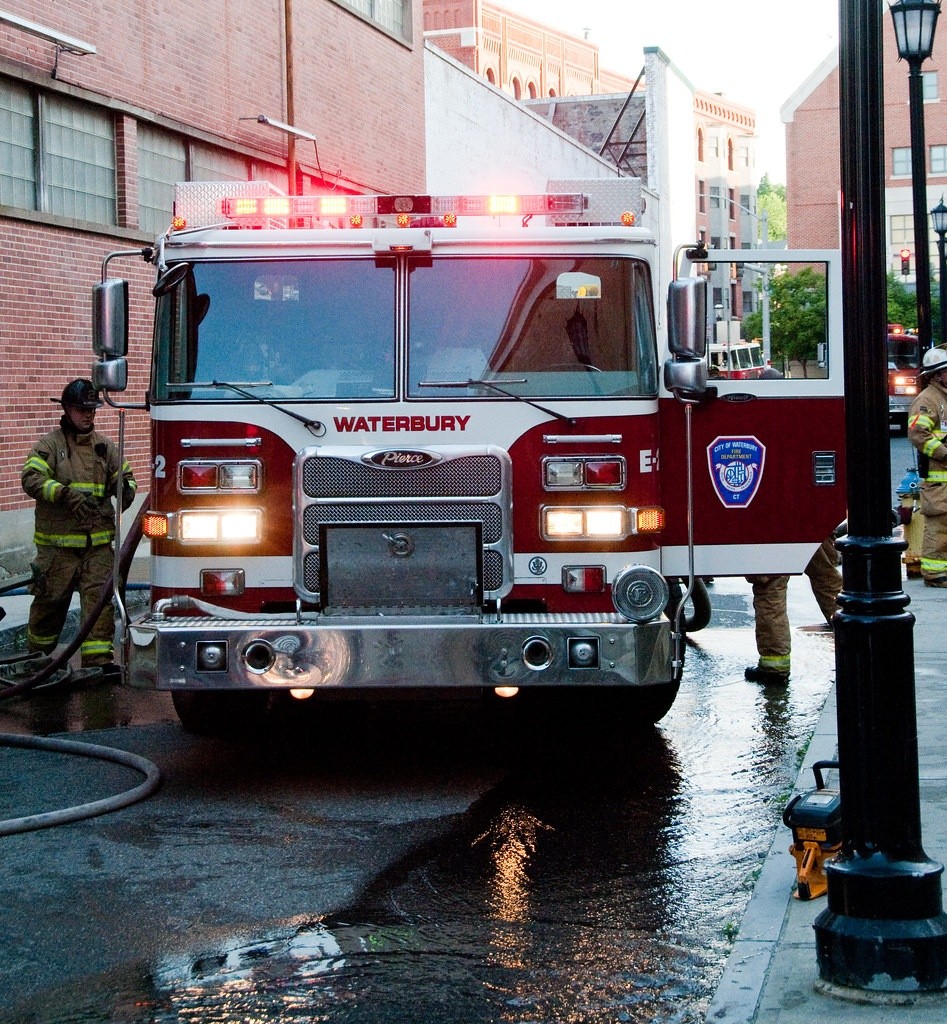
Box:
[21,379,139,677]
[908,347,947,590]
[744,527,843,683]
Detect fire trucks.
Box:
[87,179,847,730]
[707,339,766,380]
[888,323,917,434]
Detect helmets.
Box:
[917,348,947,378]
[50,379,104,408]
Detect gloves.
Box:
[113,478,135,513]
[61,487,93,519]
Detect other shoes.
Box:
[924,577,947,588]
[98,663,121,676]
[820,623,833,631]
[745,665,791,685]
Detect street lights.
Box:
[930,195,947,345]
[890,0,942,385]
[900,249,910,275]
